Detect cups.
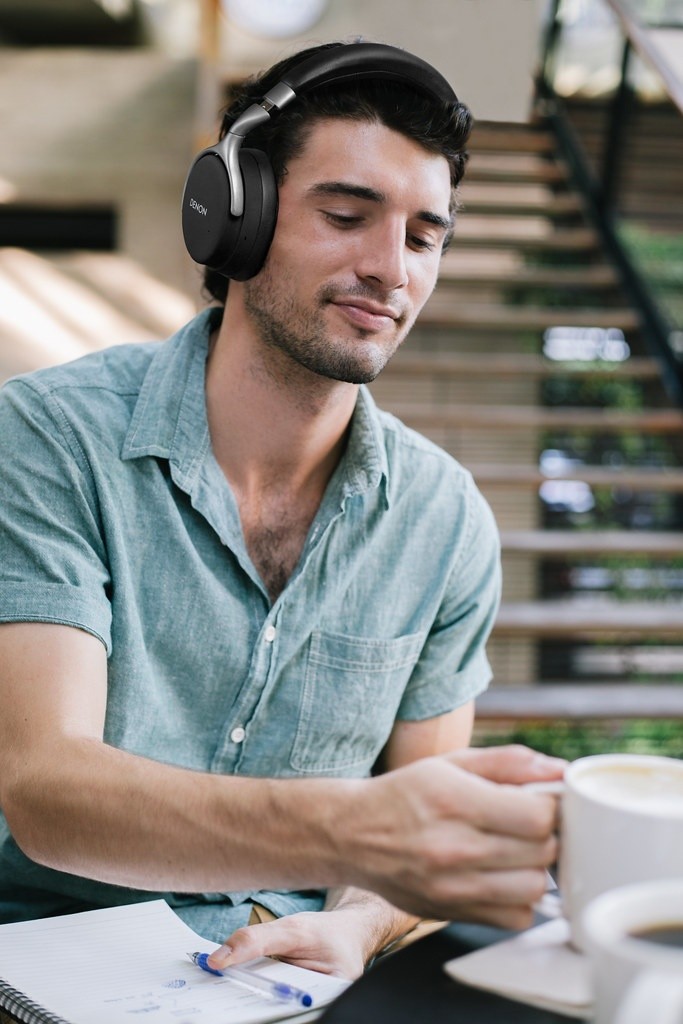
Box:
[579,888,682,1024]
[521,752,682,947]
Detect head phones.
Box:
[181,43,461,282]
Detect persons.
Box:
[3,40,573,1023]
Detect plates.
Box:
[445,921,584,1019]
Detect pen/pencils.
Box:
[185,951,313,1009]
